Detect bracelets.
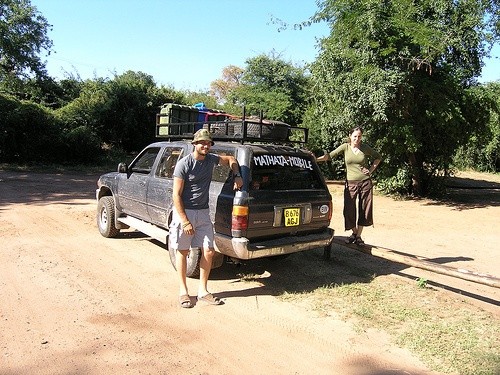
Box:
[183,221,190,227]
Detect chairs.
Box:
[159,151,181,178]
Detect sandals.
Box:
[356,236,365,247]
[345,233,357,243]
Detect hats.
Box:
[191,129,213,144]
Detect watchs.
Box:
[235,173,240,176]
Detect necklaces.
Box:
[353,148,359,152]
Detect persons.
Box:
[316,126,382,246]
[169,129,243,308]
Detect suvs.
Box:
[95,107,334,281]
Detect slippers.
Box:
[178,292,193,309]
[196,293,223,304]
